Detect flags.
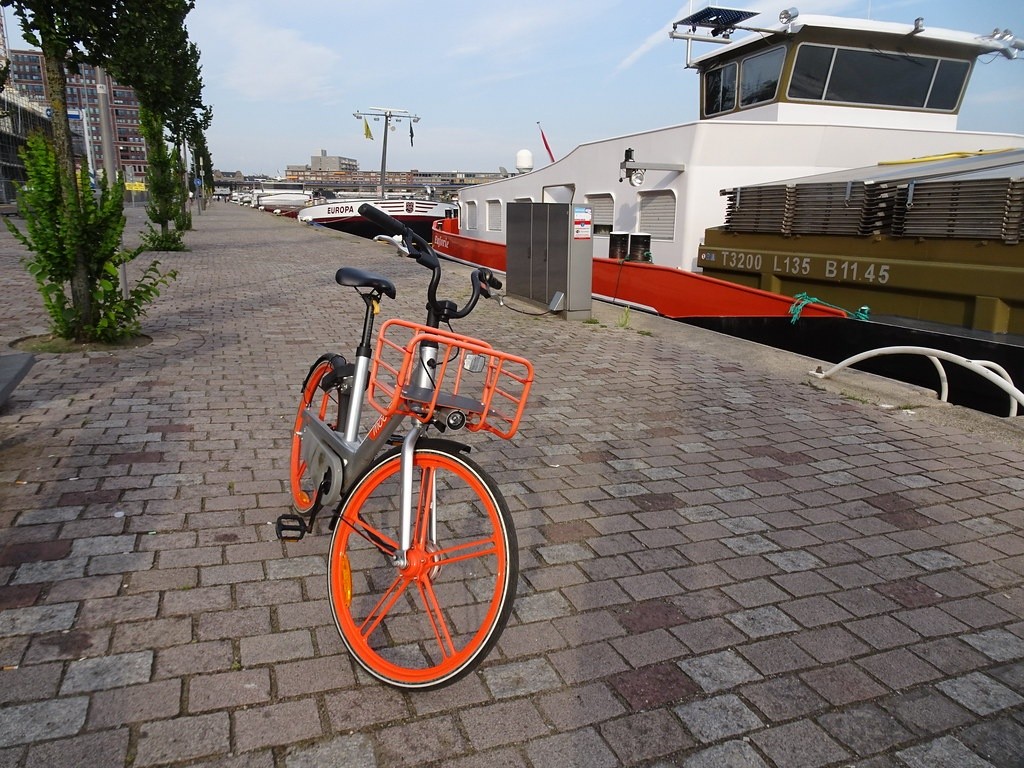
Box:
[409,120,414,147]
[364,119,374,140]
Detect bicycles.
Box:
[275,203,533,693]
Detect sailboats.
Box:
[297,105,459,224]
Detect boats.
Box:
[231,187,326,218]
[430,4,1023,383]
